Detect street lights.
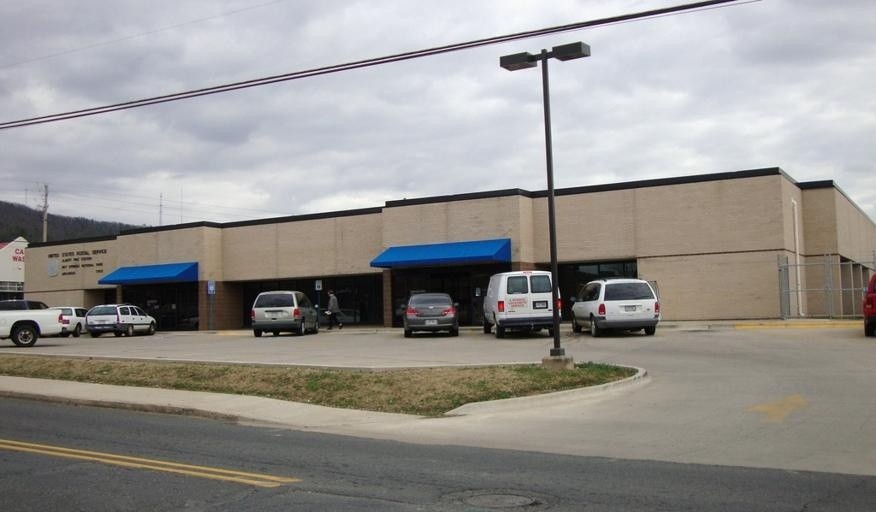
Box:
[499,42,592,357]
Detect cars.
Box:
[862,272,876,337]
[569,276,660,337]
[250,290,320,337]
[0,299,156,347]
[401,292,460,337]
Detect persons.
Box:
[327,288,343,329]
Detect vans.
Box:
[475,269,563,339]
